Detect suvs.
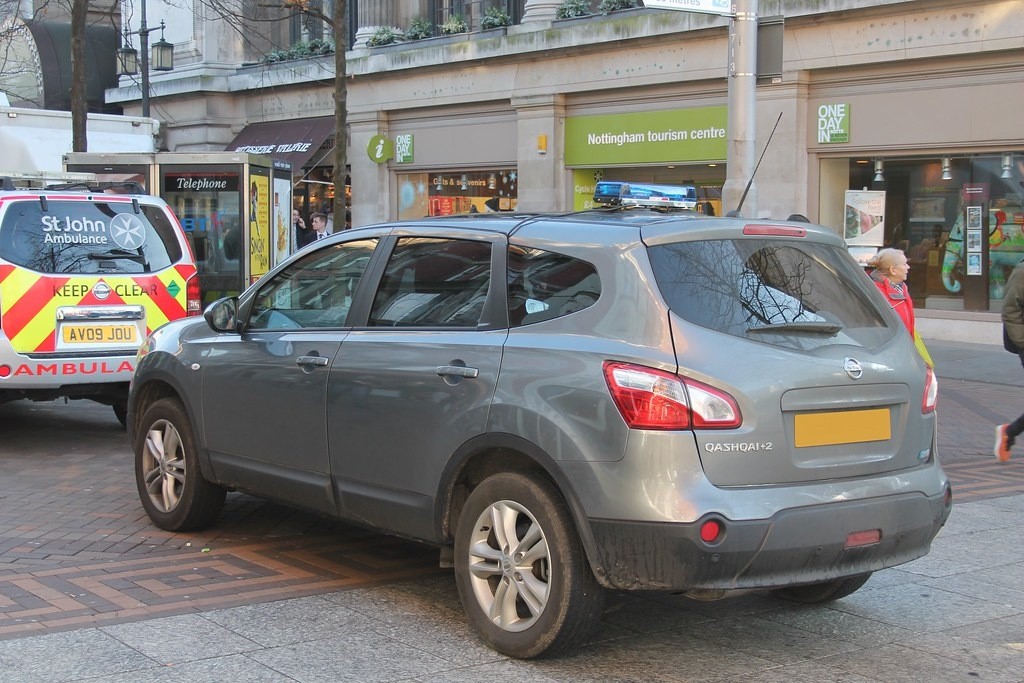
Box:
[0,171,202,435]
[124,181,954,664]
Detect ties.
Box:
[293,224,298,254]
[318,233,324,240]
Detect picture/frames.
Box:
[967,230,982,252]
[967,206,982,229]
[967,253,982,276]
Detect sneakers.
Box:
[993,424,1015,462]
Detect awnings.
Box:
[223,114,336,175]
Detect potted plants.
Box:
[596,0,644,16]
[480,5,514,30]
[437,13,470,35]
[556,0,592,19]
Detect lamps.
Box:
[941,154,953,180]
[873,157,886,182]
[488,173,497,190]
[436,175,443,190]
[460,174,469,191]
[1001,152,1014,180]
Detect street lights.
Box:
[115,1,174,119]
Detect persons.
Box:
[864,247,915,342]
[292,208,332,252]
[993,257,1024,462]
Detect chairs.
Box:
[540,257,600,319]
[397,252,480,325]
